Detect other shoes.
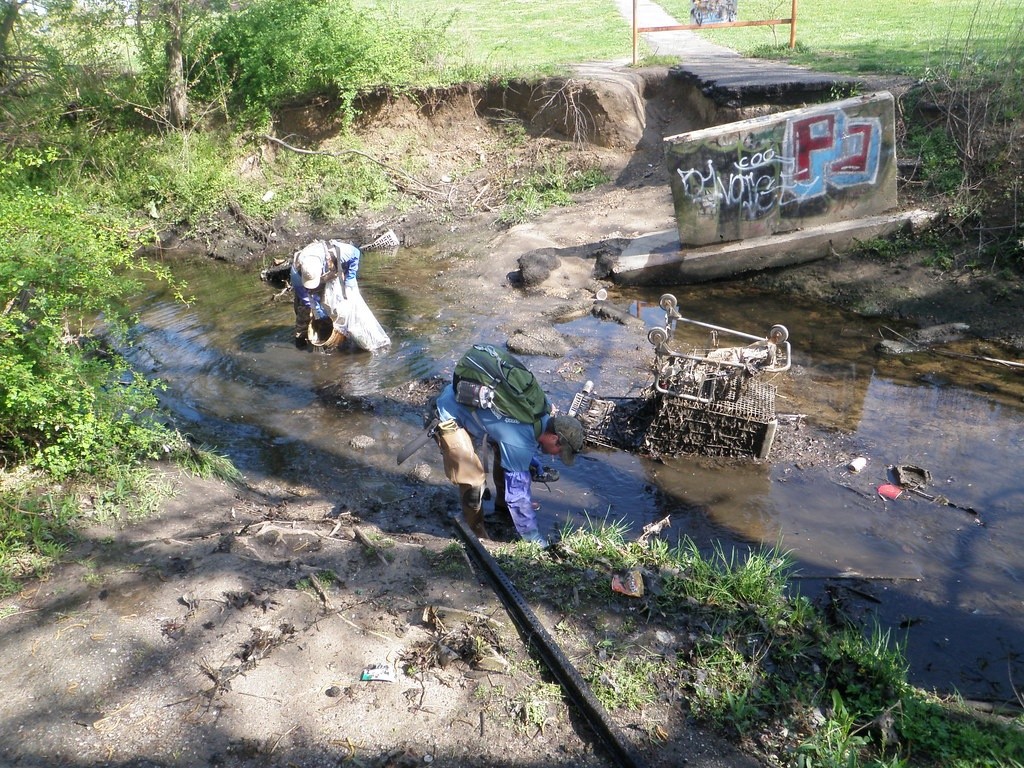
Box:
[494,487,540,510]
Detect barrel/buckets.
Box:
[308,316,345,349]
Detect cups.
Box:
[583,381,593,393]
[849,457,866,471]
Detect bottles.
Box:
[457,381,494,399]
[457,395,492,409]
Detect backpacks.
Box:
[453,344,552,439]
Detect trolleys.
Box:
[566,293,792,460]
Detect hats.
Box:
[301,256,322,289]
[555,416,584,465]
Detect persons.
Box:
[420,377,585,546]
[290,238,361,348]
